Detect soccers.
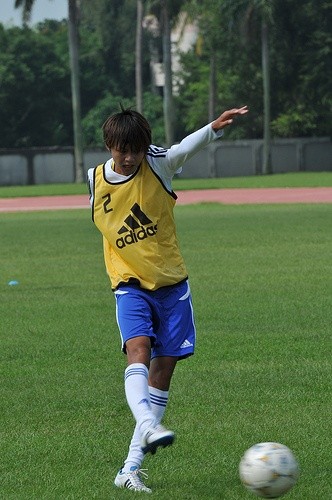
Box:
[237,441,301,499]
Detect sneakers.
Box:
[140,423,175,455]
[113,468,152,494]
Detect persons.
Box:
[88,101,249,492]
